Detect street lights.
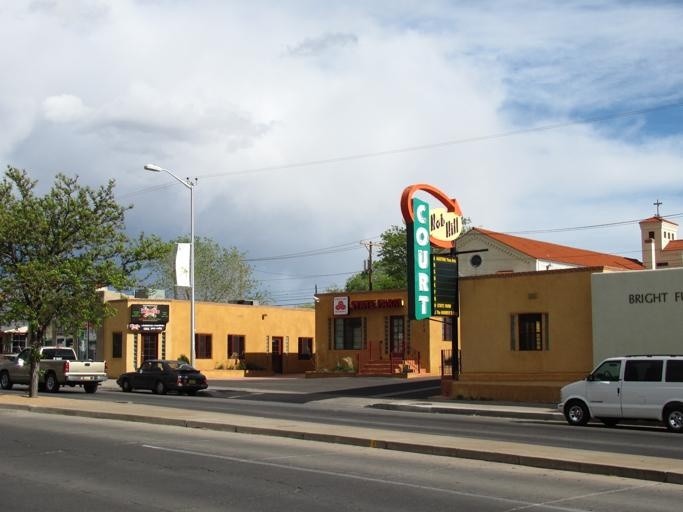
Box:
[142,164,197,369]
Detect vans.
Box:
[559,355,683,433]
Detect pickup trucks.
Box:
[0,346,107,393]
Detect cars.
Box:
[116,359,207,394]
